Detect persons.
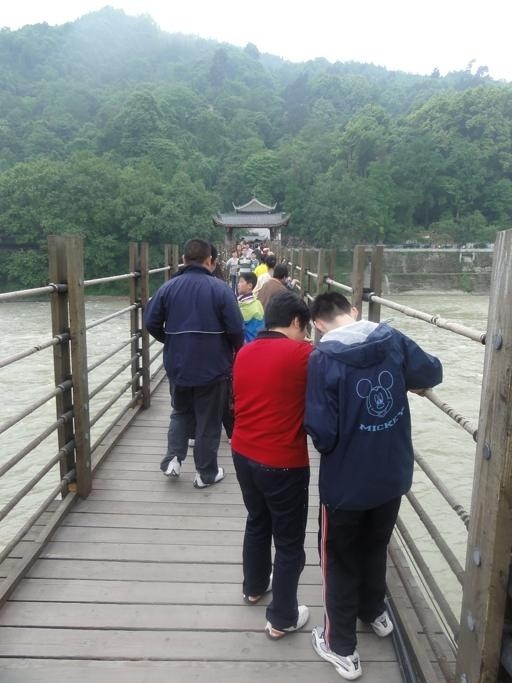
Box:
[305,291,442,681]
[237,273,265,344]
[144,239,244,489]
[256,263,289,310]
[232,289,315,642]
[238,237,276,266]
[187,244,233,448]
[226,249,238,294]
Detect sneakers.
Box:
[163,456,181,480]
[188,438,195,448]
[265,604,309,642]
[193,467,224,489]
[311,625,363,681]
[370,610,393,638]
[243,573,273,604]
